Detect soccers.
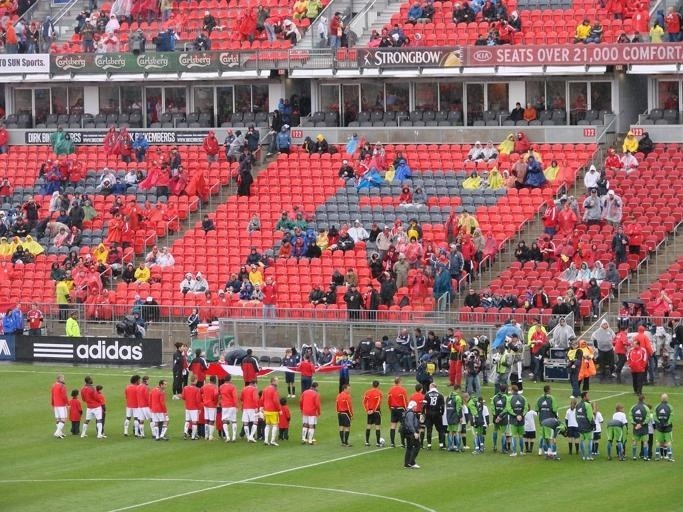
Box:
[379,438,385,445]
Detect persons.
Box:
[0,0,682,470]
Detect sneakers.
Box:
[80,434,87,437]
[366,443,431,449]
[124,432,168,441]
[97,435,106,439]
[411,464,421,469]
[61,433,66,437]
[183,432,290,443]
[55,433,63,439]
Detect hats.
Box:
[407,400,417,410]
[415,327,421,332]
[429,331,434,336]
[383,335,388,341]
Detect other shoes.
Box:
[569,451,600,461]
[607,455,675,461]
[440,445,484,454]
[494,448,560,459]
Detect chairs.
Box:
[0,105,679,135]
[2,1,679,59]
[0,138,682,330]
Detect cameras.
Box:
[505,339,509,343]
[450,338,455,343]
